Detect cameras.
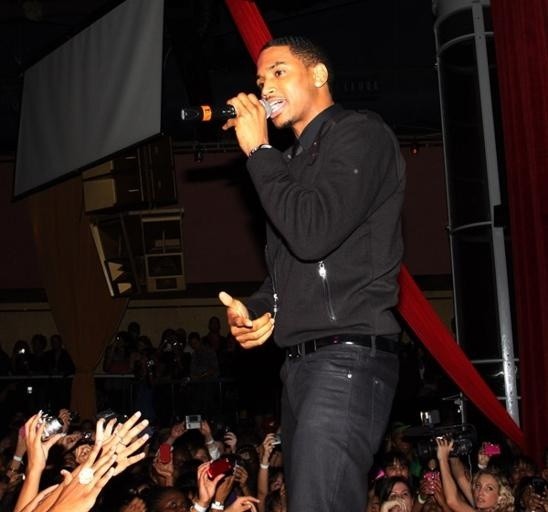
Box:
[37,414,64,442]
[270,435,281,445]
[186,415,201,429]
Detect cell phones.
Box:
[206,458,232,479]
[160,443,170,463]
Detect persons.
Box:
[217,32,407,509]
[0,316,548,510]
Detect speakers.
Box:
[81,146,138,181]
[83,172,140,215]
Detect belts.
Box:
[287,333,401,355]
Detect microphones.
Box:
[181,99,272,123]
